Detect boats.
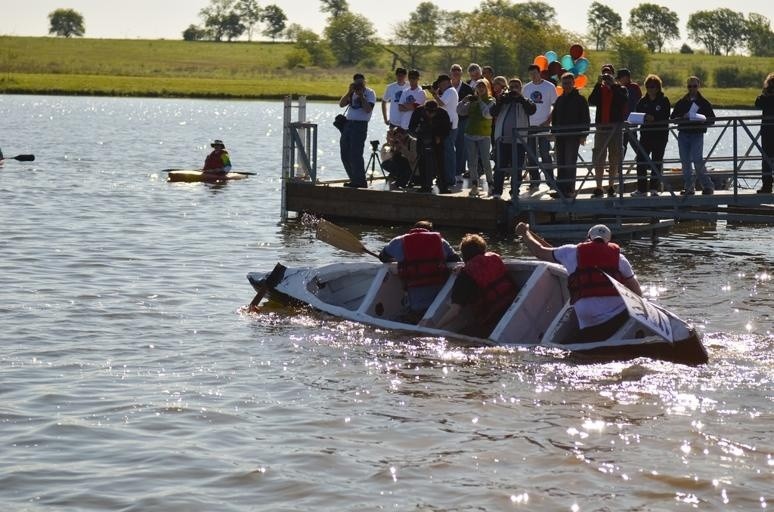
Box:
[0,157,6,169]
[246,260,710,368]
[167,170,250,183]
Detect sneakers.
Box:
[607,186,616,197]
[757,187,772,193]
[593,188,603,195]
[631,190,647,197]
[649,189,659,197]
[418,170,576,200]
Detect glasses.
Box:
[476,84,486,88]
[687,84,697,88]
[409,77,417,79]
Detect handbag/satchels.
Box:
[333,104,351,134]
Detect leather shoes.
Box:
[702,188,713,194]
[680,190,695,195]
[348,180,367,188]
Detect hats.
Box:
[587,224,612,244]
[211,140,225,149]
[601,64,614,71]
[615,70,631,79]
[437,75,451,81]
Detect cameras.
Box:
[469,96,476,101]
[422,84,431,90]
[351,82,363,91]
[502,91,511,99]
[369,140,379,146]
[601,73,611,81]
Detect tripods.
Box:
[365,153,387,183]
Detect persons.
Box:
[630,74,670,197]
[202,139,231,176]
[670,75,715,195]
[515,221,642,355]
[379,63,643,199]
[339,73,376,187]
[452,233,519,340]
[378,220,462,325]
[754,72,774,194]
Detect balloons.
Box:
[533,44,590,97]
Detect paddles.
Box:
[162,168,258,176]
[315,218,382,258]
[0,155,34,161]
